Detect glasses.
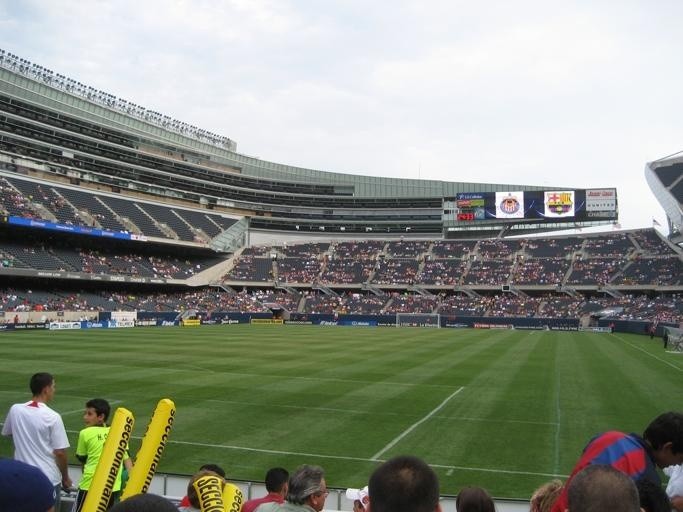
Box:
[317,488,329,496]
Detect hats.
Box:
[344,484,372,510]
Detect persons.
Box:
[0,176,105,228]
[75,398,134,511]
[0,373,72,510]
[551,412,682,510]
[106,455,682,512]
[1,229,682,330]
[0,458,56,510]
